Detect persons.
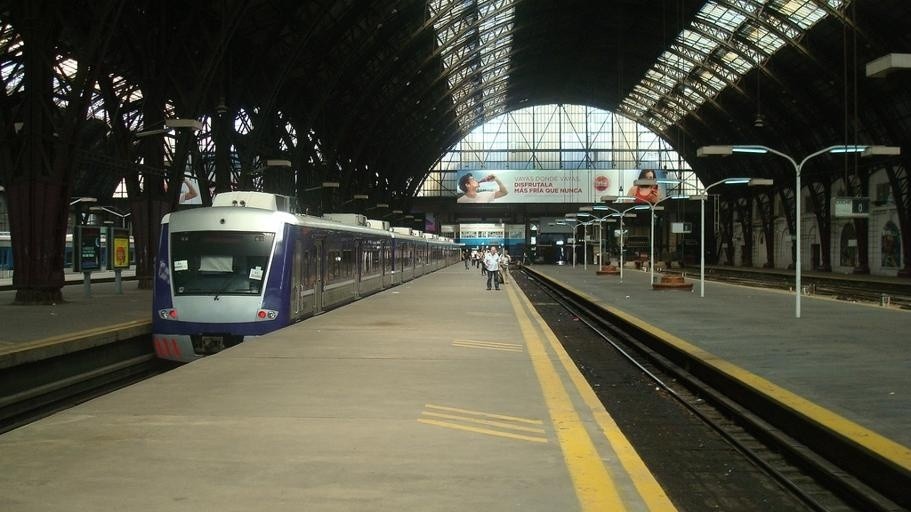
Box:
[457,173,508,203]
[625,169,658,204]
[463,245,511,290]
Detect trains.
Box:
[147,189,467,365]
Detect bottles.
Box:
[477,175,495,184]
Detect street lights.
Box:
[633,177,774,297]
[695,144,903,319]
[546,195,709,289]
